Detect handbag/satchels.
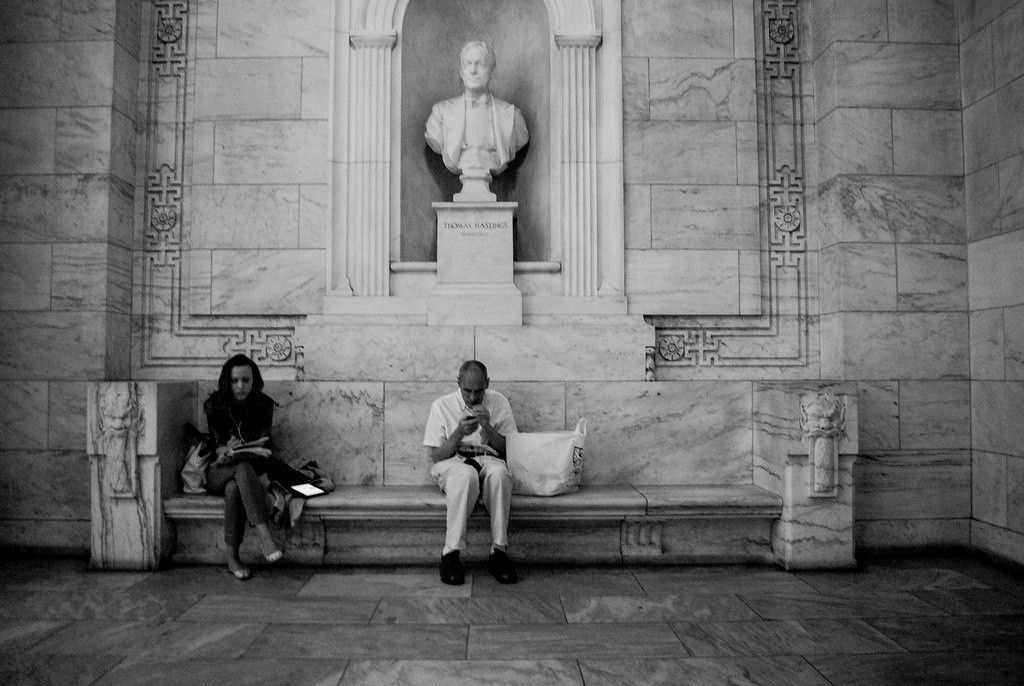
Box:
[506,417,587,496]
[181,441,213,494]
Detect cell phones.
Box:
[466,416,475,420]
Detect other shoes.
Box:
[261,543,283,562]
[229,563,250,578]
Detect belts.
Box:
[457,449,499,473]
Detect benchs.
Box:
[82,378,858,572]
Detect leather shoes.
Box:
[439,549,465,585]
[489,547,516,584]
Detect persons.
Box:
[423,361,518,586]
[422,42,531,203]
[201,353,284,579]
